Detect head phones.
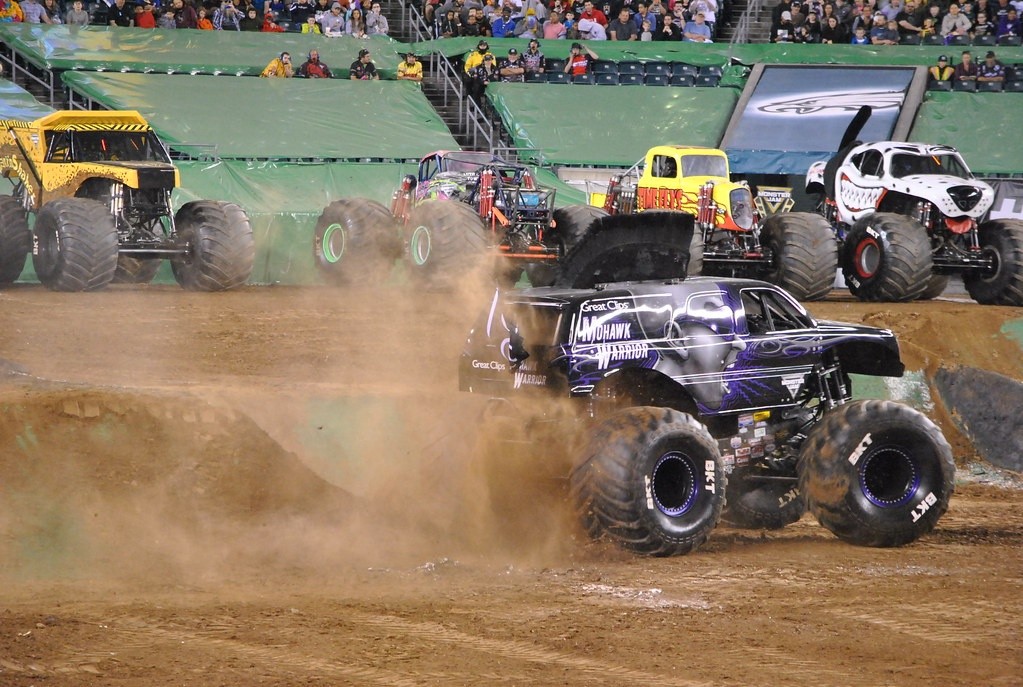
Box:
[307,52,319,60]
[280,56,291,61]
[570,44,583,52]
[528,42,541,48]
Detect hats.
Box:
[938,55,947,62]
[332,2,341,9]
[697,11,705,17]
[572,43,580,48]
[530,38,537,42]
[791,1,801,7]
[782,11,792,20]
[509,48,517,54]
[986,51,995,58]
[502,6,511,13]
[479,40,487,45]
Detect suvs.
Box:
[0,107,253,294]
[460,276,958,559]
[804,140,1023,302]
[313,148,610,295]
[601,142,837,300]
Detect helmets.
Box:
[805,161,827,194]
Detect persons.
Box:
[928,55,956,92]
[636,282,747,411]
[955,51,978,81]
[523,38,546,73]
[976,51,1006,82]
[472,54,500,123]
[0,0,389,34]
[299,48,334,78]
[350,49,380,80]
[665,156,676,177]
[564,42,599,81]
[407,0,732,41]
[396,52,425,91]
[464,41,496,99]
[261,51,294,78]
[498,48,527,83]
[767,0,1023,47]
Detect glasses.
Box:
[906,6,914,8]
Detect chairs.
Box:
[573,73,595,85]
[923,34,944,46]
[695,75,718,87]
[544,60,565,72]
[64,3,74,14]
[699,65,724,77]
[618,62,645,77]
[619,73,643,85]
[644,62,671,77]
[595,73,619,85]
[671,61,697,77]
[88,2,109,22]
[670,74,695,87]
[644,73,669,86]
[549,72,571,84]
[592,61,618,75]
[525,70,547,83]
[949,35,1021,46]
[898,33,921,45]
[929,62,1023,93]
